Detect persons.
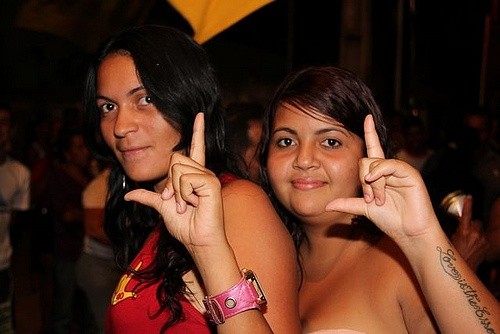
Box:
[31,130,104,334]
[78,20,304,334]
[0,105,33,334]
[384,96,500,302]
[255,66,500,333]
[213,98,268,189]
[76,143,115,333]
[22,108,72,217]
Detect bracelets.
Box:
[202,268,270,325]
